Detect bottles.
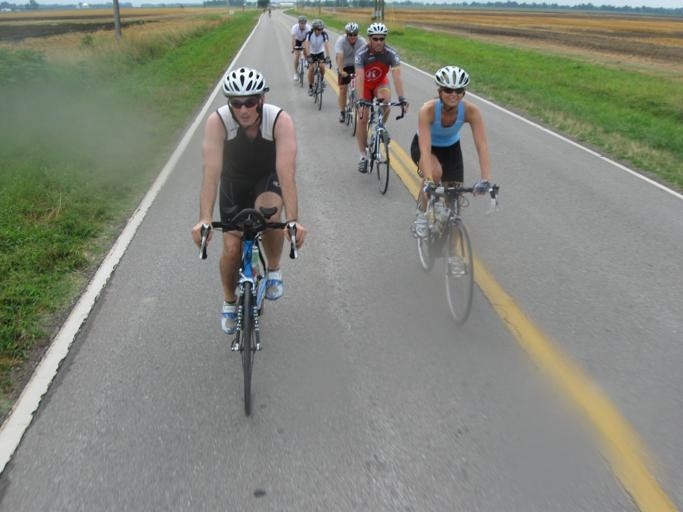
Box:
[251,247,260,278]
[437,208,450,228]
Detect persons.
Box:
[290,16,312,82]
[333,21,368,124]
[267,8,271,17]
[187,67,308,335]
[352,21,411,176]
[409,63,495,281]
[303,19,331,98]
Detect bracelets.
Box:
[284,218,296,224]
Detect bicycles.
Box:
[307,57,332,110]
[410,182,501,326]
[293,45,307,87]
[342,72,358,137]
[196,206,299,419]
[353,97,408,194]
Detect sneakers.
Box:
[293,70,300,81]
[308,88,313,96]
[265,268,284,300]
[357,156,368,173]
[221,299,239,335]
[338,111,345,122]
[415,209,430,238]
[448,255,465,278]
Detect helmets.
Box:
[310,19,324,31]
[434,65,470,89]
[221,66,266,97]
[365,21,388,35]
[297,15,307,24]
[344,22,360,34]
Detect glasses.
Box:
[230,98,257,109]
[441,87,464,94]
[347,33,358,37]
[372,37,385,41]
